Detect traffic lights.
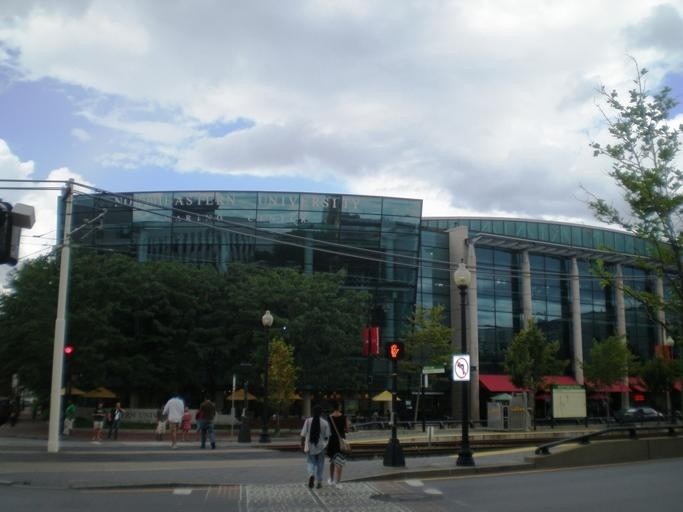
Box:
[65,344,73,356]
[386,341,405,360]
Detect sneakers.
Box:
[327,478,335,486]
[309,476,315,489]
[91,436,119,441]
[200,443,217,450]
[169,445,177,449]
[316,482,323,489]
[336,483,343,490]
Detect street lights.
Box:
[453,258,475,465]
[261,308,273,441]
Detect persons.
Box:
[152,404,172,443]
[196,392,218,448]
[386,409,391,422]
[31,397,37,422]
[60,402,78,436]
[161,389,184,444]
[369,411,379,422]
[194,409,203,444]
[299,404,331,489]
[180,404,191,441]
[101,401,128,441]
[89,401,107,441]
[321,400,347,491]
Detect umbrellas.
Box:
[59,386,87,396]
[79,387,116,398]
[267,388,374,400]
[227,388,257,401]
[371,391,401,402]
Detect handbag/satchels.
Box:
[338,437,353,454]
[303,431,310,453]
[195,410,205,422]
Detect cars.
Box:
[616,407,664,419]
